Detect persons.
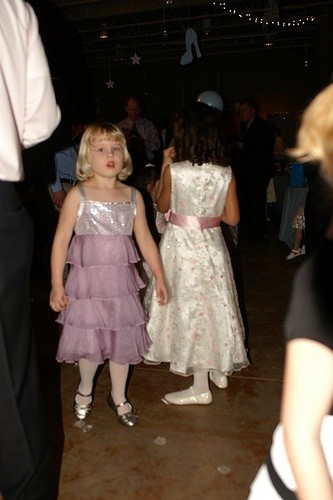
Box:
[143,122,250,406]
[228,100,283,242]
[50,120,169,431]
[0,0,65,500]
[46,95,309,262]
[246,82,333,500]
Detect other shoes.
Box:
[208,370,229,389]
[286,248,303,260]
[108,393,139,427]
[73,383,93,420]
[164,385,213,405]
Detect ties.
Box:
[132,124,137,133]
